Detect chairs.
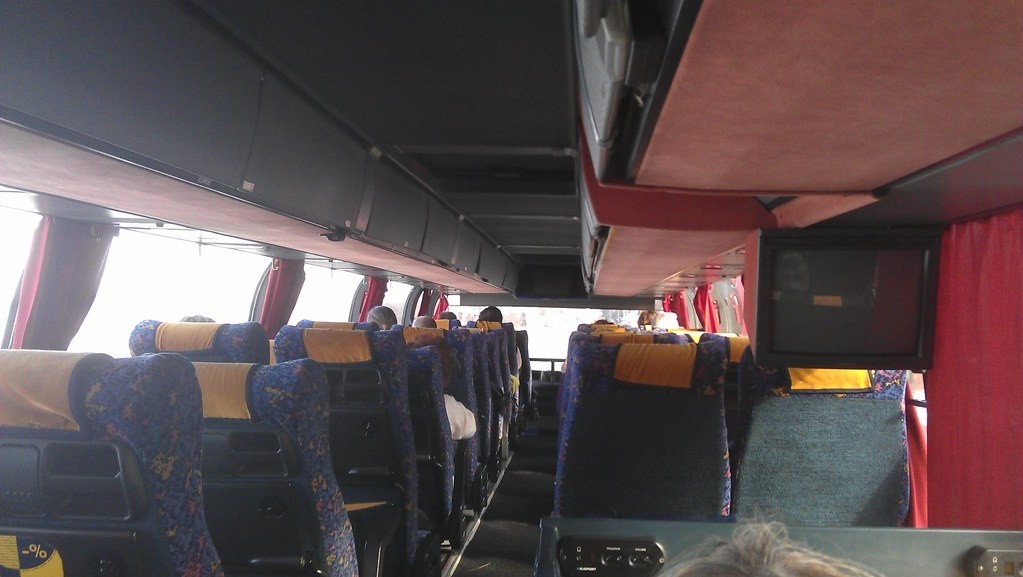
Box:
[0,317,913,577]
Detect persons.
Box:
[479,306,522,370]
[653,519,880,577]
[412,316,437,328]
[406,333,477,440]
[637,311,662,327]
[179,315,216,322]
[438,311,457,320]
[367,306,397,330]
